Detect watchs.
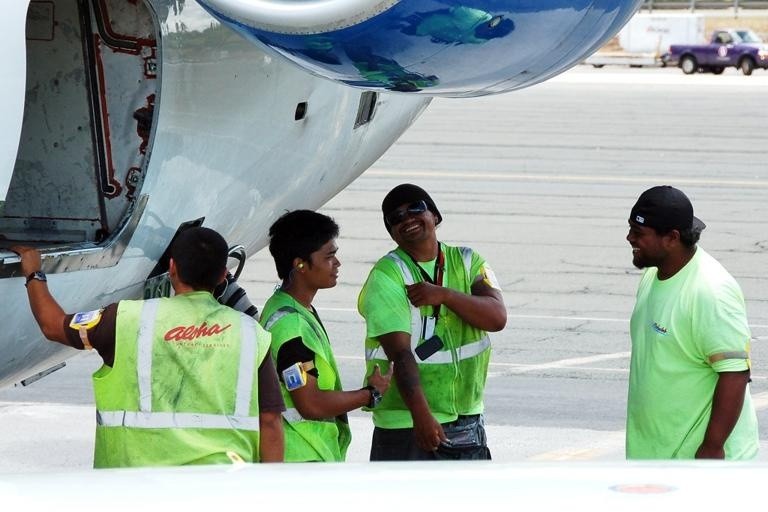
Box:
[359,386,383,410]
[23,269,48,288]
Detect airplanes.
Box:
[0,0,647,393]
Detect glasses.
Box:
[387,199,428,227]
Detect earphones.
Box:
[297,262,305,272]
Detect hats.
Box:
[629,185,706,234]
[382,184,442,226]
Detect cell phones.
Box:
[415,334,445,362]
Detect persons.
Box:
[256,209,396,463]
[11,226,287,467]
[382,2,517,49]
[358,182,508,460]
[342,37,444,94]
[282,34,342,65]
[624,184,763,460]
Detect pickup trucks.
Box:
[669,28,767,75]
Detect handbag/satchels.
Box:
[432,414,488,459]
